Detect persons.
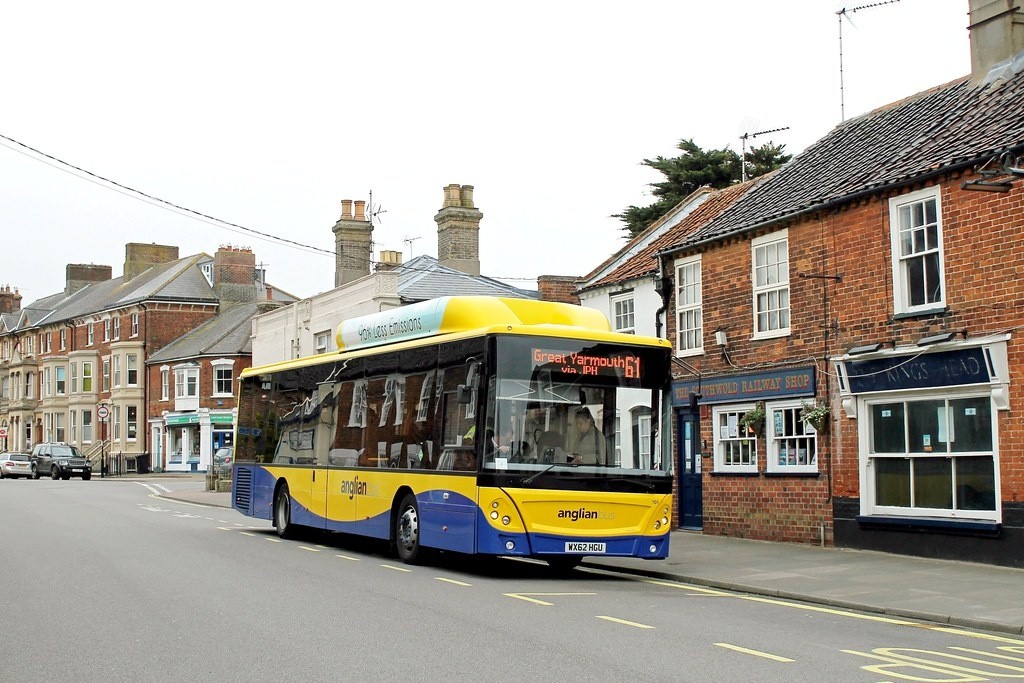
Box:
[572,407,606,466]
[284,398,297,411]
[358,397,386,467]
[485,417,513,462]
[176,437,182,452]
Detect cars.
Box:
[0,453,34,479]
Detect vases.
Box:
[749,420,762,439]
[808,416,826,436]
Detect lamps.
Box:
[961,151,1024,193]
[917,330,967,347]
[218,400,223,406]
[848,341,896,356]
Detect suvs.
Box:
[215,447,234,465]
[31,443,94,480]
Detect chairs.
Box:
[537,430,563,460]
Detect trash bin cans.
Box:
[136,453,150,474]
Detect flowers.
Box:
[736,404,765,433]
[798,397,831,431]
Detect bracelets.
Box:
[581,455,582,464]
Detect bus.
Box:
[229,294,703,573]
